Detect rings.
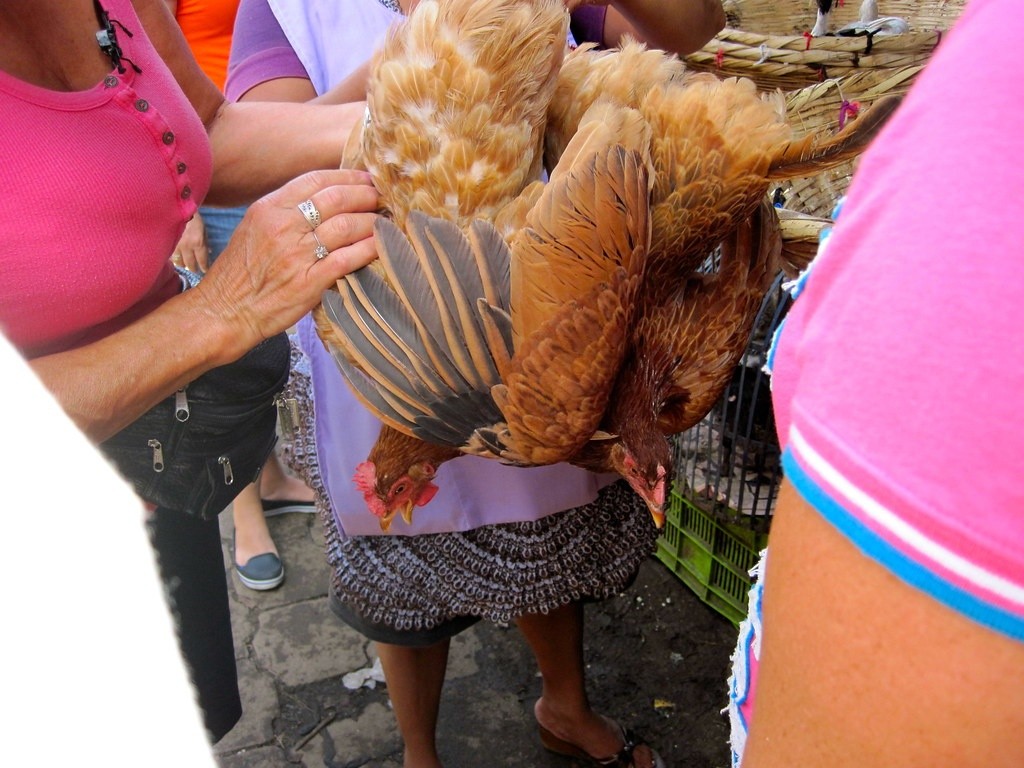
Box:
[172,255,181,261]
[298,199,321,229]
[312,232,328,260]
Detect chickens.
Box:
[803,0,909,37]
[697,288,795,486]
[310,31,907,528]
[337,0,573,536]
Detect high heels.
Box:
[536,706,666,768]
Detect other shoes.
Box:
[258,498,320,517]
[233,525,285,591]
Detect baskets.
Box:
[649,480,770,628]
[665,0,967,524]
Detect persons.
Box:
[730,0,1024,768]
[0,0,370,743]
[168,1,321,591]
[223,0,727,768]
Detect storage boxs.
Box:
[655,482,773,627]
